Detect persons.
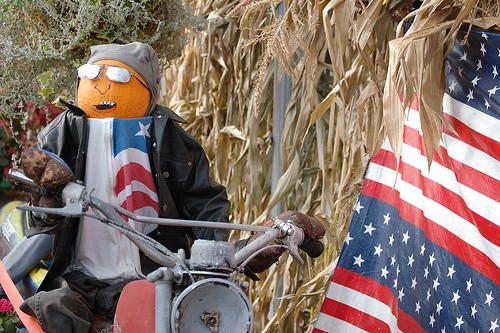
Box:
[19,42,326,332]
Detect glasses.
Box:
[78,64,150,93]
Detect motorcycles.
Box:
[0,142,326,331]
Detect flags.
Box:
[311,30,500,333]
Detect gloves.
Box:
[245,210,325,273]
[22,148,74,228]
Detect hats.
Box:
[76,41,160,117]
